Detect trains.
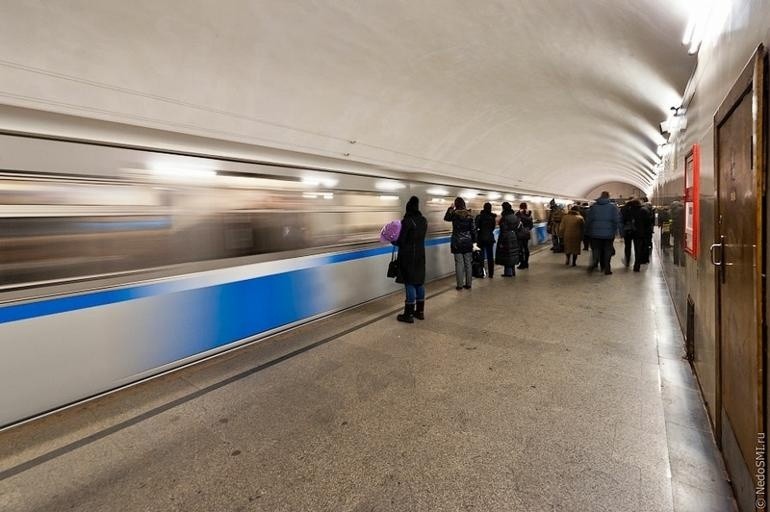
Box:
[1,101,597,431]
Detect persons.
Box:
[391,195,426,324]
[474,202,495,278]
[443,197,476,290]
[514,202,534,269]
[658,198,689,267]
[495,202,519,277]
[543,190,657,276]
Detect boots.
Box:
[397,301,415,323]
[414,299,424,320]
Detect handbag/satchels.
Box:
[387,260,398,278]
[473,264,484,277]
[517,228,530,240]
[621,221,637,233]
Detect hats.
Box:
[406,196,419,213]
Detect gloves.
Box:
[473,242,481,252]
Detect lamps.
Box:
[660,104,688,135]
